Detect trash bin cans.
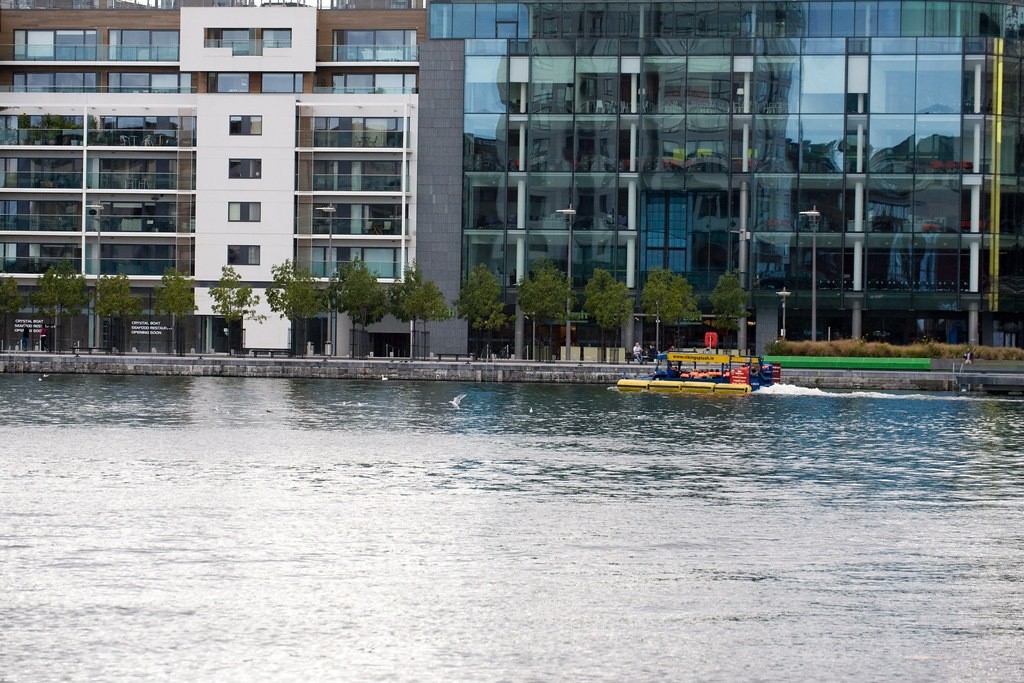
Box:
[324,340,332,360]
[306,341,315,356]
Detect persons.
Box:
[632,342,644,363]
[39,323,48,351]
[963,347,973,364]
[669,364,748,379]
[668,346,674,351]
[704,347,712,354]
[22,325,29,351]
[648,345,658,360]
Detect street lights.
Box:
[314,207,333,357]
[85,203,104,347]
[554,204,576,361]
[799,205,820,342]
[776,287,792,342]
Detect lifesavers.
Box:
[750,365,760,376]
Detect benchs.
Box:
[436,354,478,361]
[69,347,111,355]
[251,349,293,358]
[626,357,654,365]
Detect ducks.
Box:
[43,374,49,378]
[381,375,388,380]
[529,407,533,412]
[38,378,42,381]
[343,400,351,405]
[357,401,368,407]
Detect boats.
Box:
[616,352,753,394]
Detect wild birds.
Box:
[449,394,467,409]
